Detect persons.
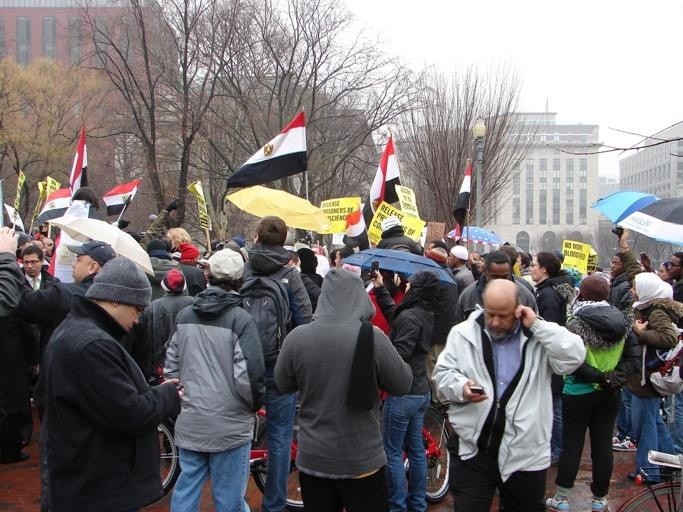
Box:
[18,233,30,253]
[20,240,114,367]
[449,245,475,299]
[149,238,168,264]
[335,248,353,269]
[425,246,464,388]
[168,229,194,245]
[434,279,587,512]
[39,257,186,512]
[374,216,424,255]
[608,252,633,307]
[34,239,46,258]
[516,251,537,291]
[474,252,489,282]
[426,247,448,270]
[426,241,445,255]
[500,245,535,294]
[0,226,20,339]
[283,247,320,305]
[20,250,53,428]
[621,225,683,482]
[164,249,267,511]
[656,260,671,288]
[179,243,207,291]
[466,250,481,271]
[669,250,683,314]
[452,253,539,325]
[375,268,440,512]
[128,199,179,252]
[548,276,616,512]
[530,252,573,495]
[239,215,313,512]
[43,238,54,255]
[142,268,197,360]
[277,269,413,512]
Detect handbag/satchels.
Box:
[346,321,375,412]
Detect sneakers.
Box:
[611,433,625,443]
[546,496,569,512]
[591,498,607,511]
[614,437,641,454]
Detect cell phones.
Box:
[471,385,484,394]
[176,382,183,390]
[370,262,379,276]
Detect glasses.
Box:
[669,263,681,267]
[611,262,622,266]
[531,263,542,267]
[136,307,145,316]
[25,259,41,264]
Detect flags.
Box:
[367,137,402,224]
[68,127,94,196]
[224,107,314,186]
[454,158,471,233]
[103,179,145,214]
[342,210,372,249]
[36,190,71,221]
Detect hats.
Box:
[17,231,31,244]
[68,242,114,265]
[84,257,153,305]
[180,242,199,260]
[209,248,244,283]
[161,270,186,293]
[579,276,610,301]
[428,247,448,263]
[381,216,402,231]
[451,245,469,261]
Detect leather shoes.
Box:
[629,471,659,485]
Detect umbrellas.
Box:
[620,197,683,249]
[447,226,504,247]
[345,246,457,286]
[225,185,333,236]
[49,213,155,277]
[591,190,655,237]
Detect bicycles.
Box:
[615,450,683,512]
[250,406,451,511]
[159,419,180,491]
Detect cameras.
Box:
[612,226,623,237]
